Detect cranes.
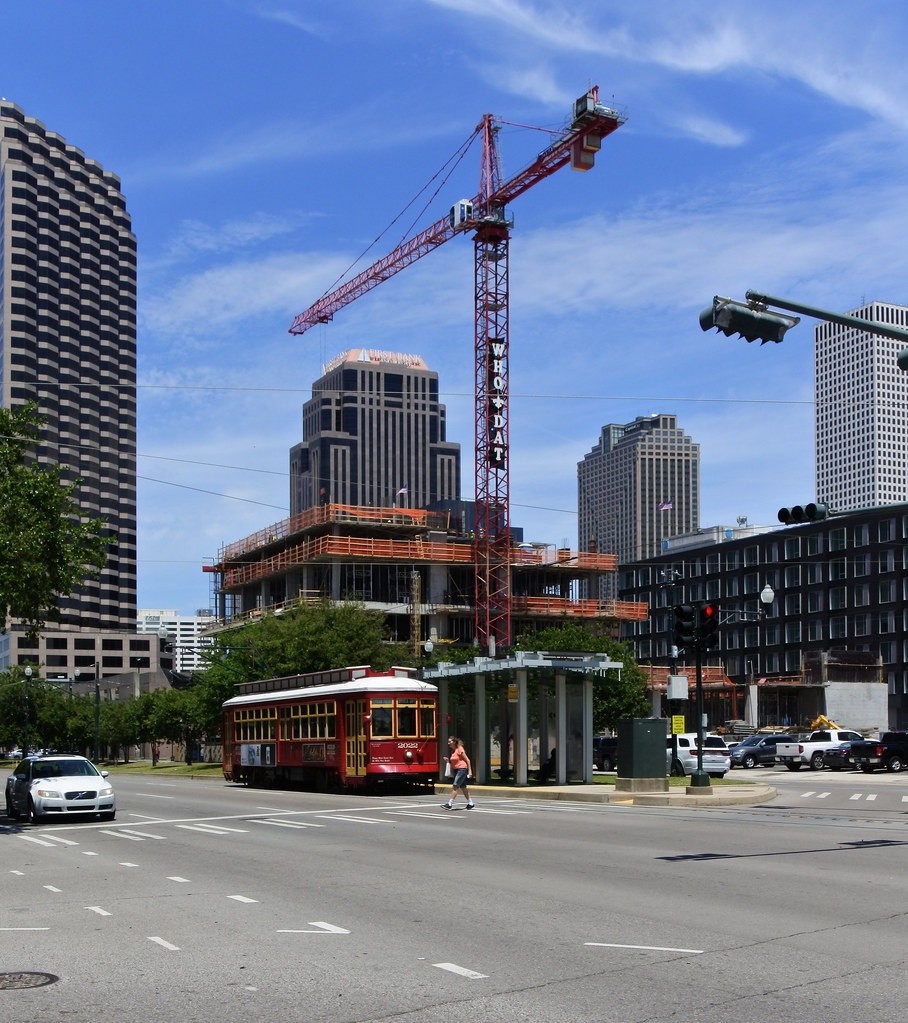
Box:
[409,571,420,659]
[289,85,627,649]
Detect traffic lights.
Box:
[777,503,828,524]
[674,606,696,648]
[699,296,801,346]
[697,602,719,648]
[898,348,908,372]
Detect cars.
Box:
[8,749,42,760]
[821,741,881,771]
[666,733,730,779]
[728,734,797,769]
[593,736,618,771]
[38,749,50,754]
[716,720,741,748]
[6,754,116,823]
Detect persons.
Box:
[400,716,415,734]
[440,736,474,810]
[533,748,556,784]
[378,720,389,732]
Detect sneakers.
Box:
[463,803,475,810]
[440,802,453,811]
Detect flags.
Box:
[657,501,673,510]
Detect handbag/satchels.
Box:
[444,760,455,778]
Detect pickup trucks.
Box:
[844,731,908,773]
[775,729,881,771]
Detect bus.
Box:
[222,665,439,794]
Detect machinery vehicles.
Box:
[810,715,842,730]
